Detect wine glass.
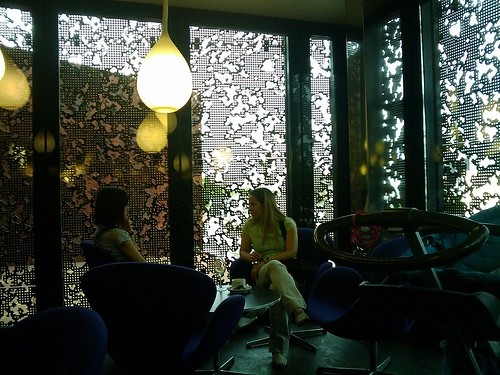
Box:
[214,256,226,291]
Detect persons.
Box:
[93,186,149,265]
[240,188,311,368]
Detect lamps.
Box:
[136,0,194,113]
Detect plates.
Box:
[227,286,252,293]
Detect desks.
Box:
[194,284,281,375]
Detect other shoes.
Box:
[292,308,310,326]
[272,351,287,367]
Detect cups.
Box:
[232,278,246,290]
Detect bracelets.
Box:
[262,260,267,264]
[266,257,270,262]
[129,230,135,237]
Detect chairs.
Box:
[80,262,246,375]
[82,239,118,269]
[0,306,107,375]
[306,236,411,375]
[404,206,500,375]
[229,228,334,353]
[368,239,412,281]
